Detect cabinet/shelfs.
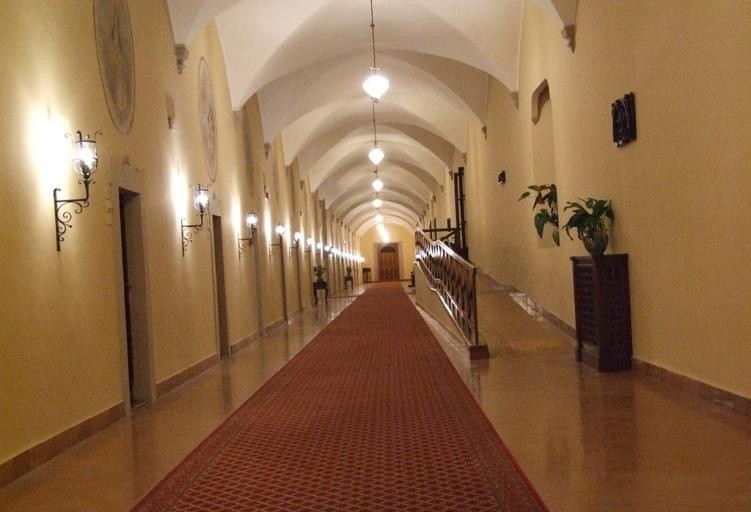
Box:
[570,253,631,373]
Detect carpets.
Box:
[131,280,547,512]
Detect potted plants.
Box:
[313,264,327,281]
[563,196,616,256]
[346,266,353,275]
[519,182,561,247]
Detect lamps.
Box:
[371,166,383,191]
[368,96,386,165]
[238,212,260,261]
[53,129,106,251]
[271,225,312,256]
[361,0,391,102]
[181,184,211,258]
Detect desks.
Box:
[313,281,329,304]
[345,277,354,289]
